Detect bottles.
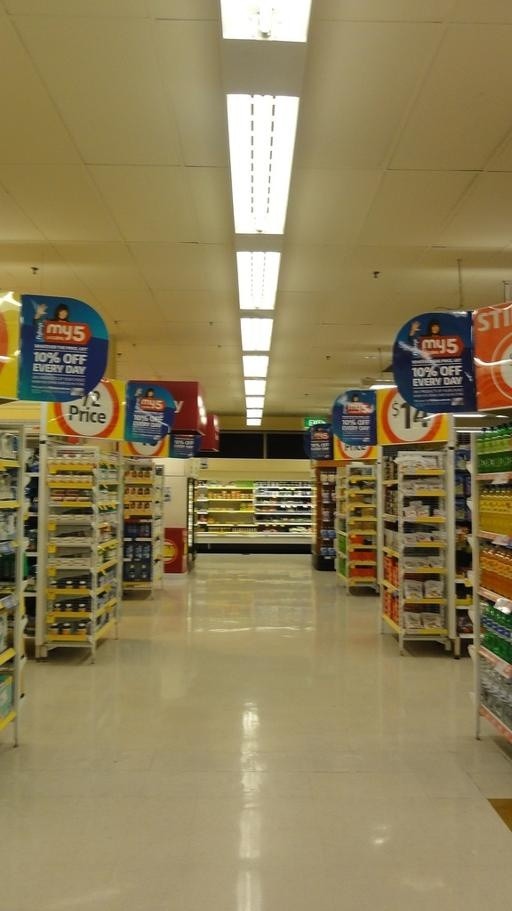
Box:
[47,572,121,643]
[477,423,512,733]
[123,520,154,581]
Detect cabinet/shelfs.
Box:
[186,475,315,550]
[126,444,166,609]
[316,463,335,570]
[28,438,125,664]
[475,414,512,750]
[382,435,474,656]
[337,457,381,594]
[1,422,26,748]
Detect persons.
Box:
[352,394,360,403]
[409,319,441,341]
[135,388,154,399]
[33,303,71,329]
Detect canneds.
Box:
[49,580,88,636]
